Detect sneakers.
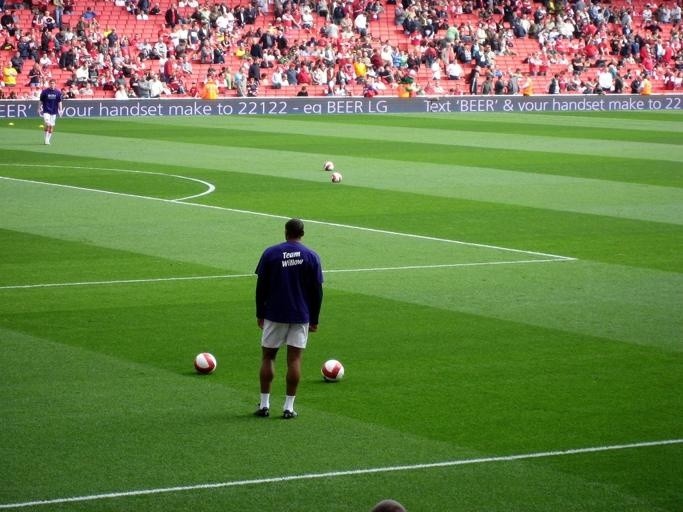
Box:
[255,407,270,417]
[283,409,297,418]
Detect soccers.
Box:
[324,360,344,382]
[196,353,218,375]
[326,162,333,171]
[332,174,342,183]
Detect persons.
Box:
[39,79,63,146]
[164,1,354,98]
[511,1,682,99]
[253,218,324,419]
[1,2,172,100]
[333,1,534,98]
